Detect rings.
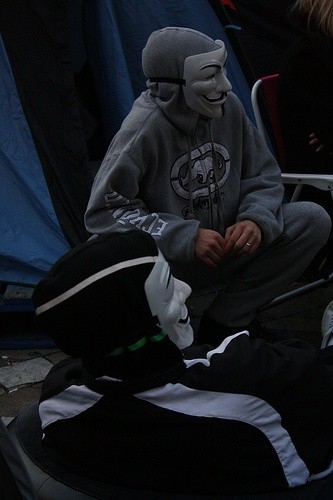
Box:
[246,242,252,249]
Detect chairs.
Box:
[251,74,333,203]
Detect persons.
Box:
[83,26,331,350]
[275,0,333,282]
[30,229,333,488]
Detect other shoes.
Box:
[196,311,287,349]
